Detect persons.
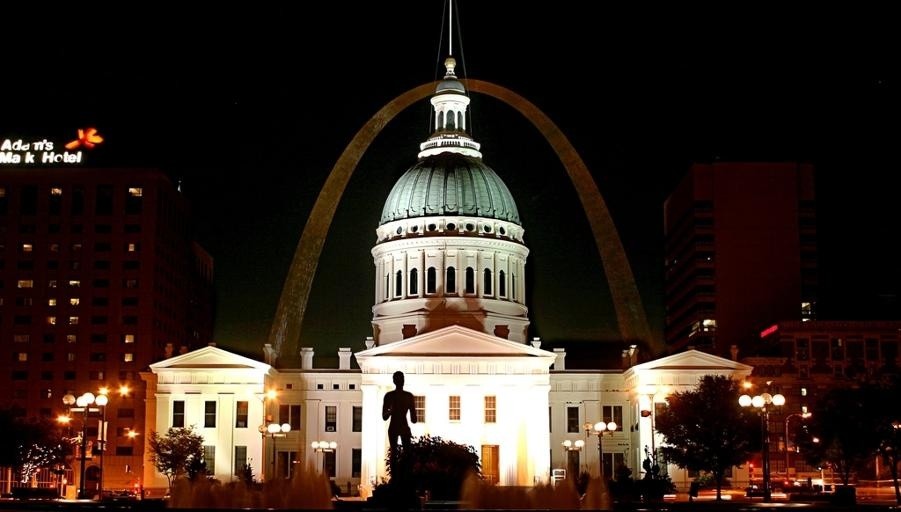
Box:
[382,369,419,476]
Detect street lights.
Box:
[635,385,669,452]
[582,422,617,480]
[738,392,786,498]
[785,414,812,478]
[62,392,108,500]
[258,422,291,480]
[312,441,337,474]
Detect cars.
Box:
[747,479,836,496]
